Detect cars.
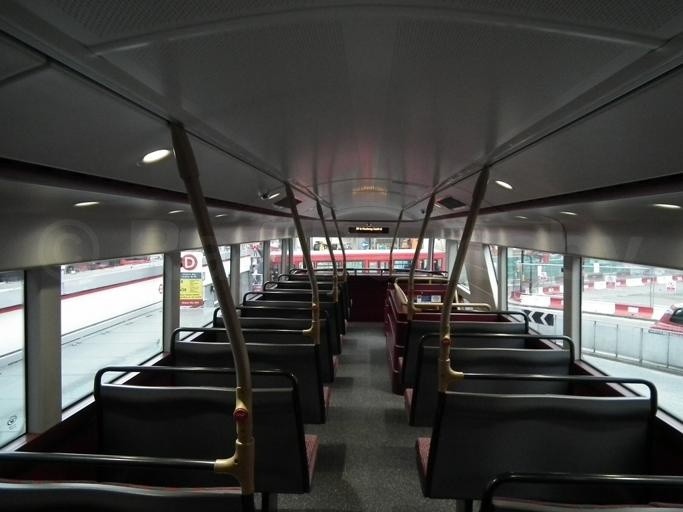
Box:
[645,304,683,337]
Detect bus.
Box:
[248,247,446,292]
[1,242,251,361]
